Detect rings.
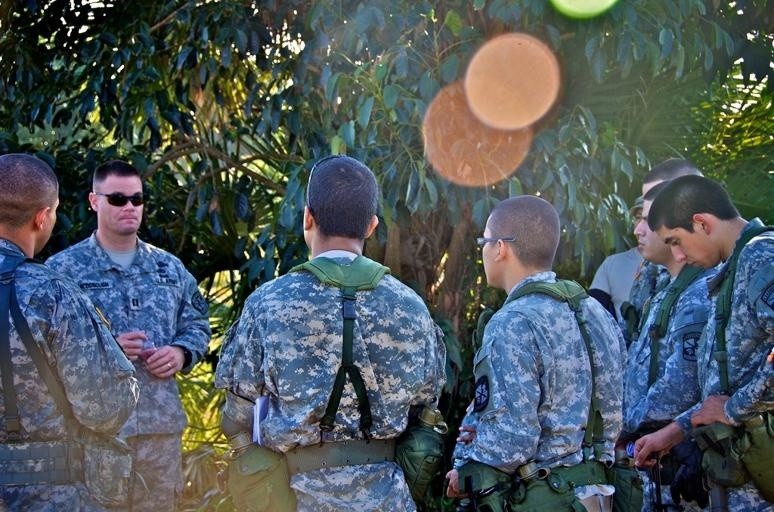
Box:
[166,361,173,369]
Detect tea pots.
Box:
[227,429,256,460]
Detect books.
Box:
[251,395,268,447]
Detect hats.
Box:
[629,194,646,217]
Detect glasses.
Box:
[93,191,147,208]
[305,153,347,224]
[474,235,516,247]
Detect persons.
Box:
[444,194,631,512]
[213,152,447,512]
[632,174,773,512]
[588,190,650,355]
[1,151,140,511]
[43,160,213,511]
[611,156,722,512]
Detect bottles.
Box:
[415,406,451,436]
[613,447,637,467]
[517,459,553,485]
[741,409,770,434]
